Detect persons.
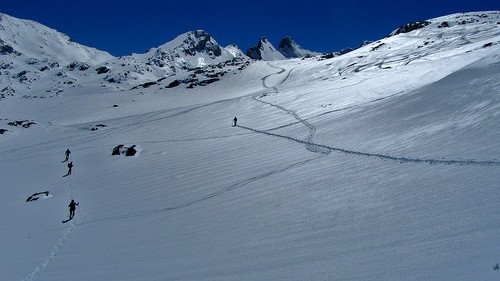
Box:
[234,117,237,126]
[68,200,79,218]
[68,162,73,175]
[65,149,71,160]
